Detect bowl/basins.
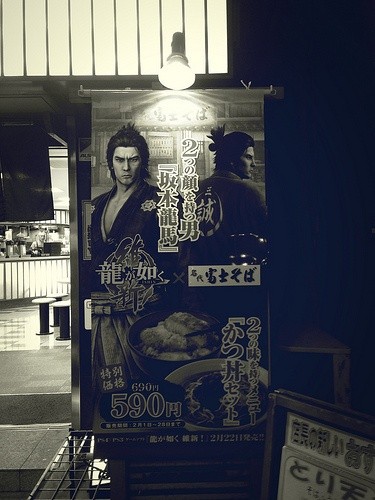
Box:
[126,309,225,378]
[164,358,269,431]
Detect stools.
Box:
[51,301,70,340]
[31,297,56,335]
[48,294,68,327]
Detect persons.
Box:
[30,233,46,254]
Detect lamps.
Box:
[157,32,195,90]
